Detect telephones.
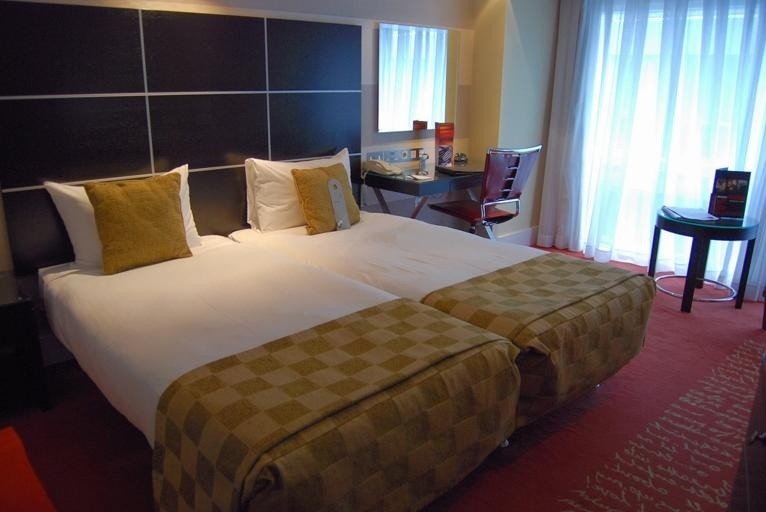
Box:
[362,159,402,175]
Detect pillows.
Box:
[82,172,193,275]
[43,161,204,267]
[244,147,353,233]
[291,162,362,235]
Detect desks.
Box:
[646,205,761,313]
[365,161,497,240]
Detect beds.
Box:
[227,206,658,429]
[37,232,525,510]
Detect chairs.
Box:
[427,143,543,240]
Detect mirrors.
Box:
[376,21,448,132]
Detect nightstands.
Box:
[0,272,54,420]
